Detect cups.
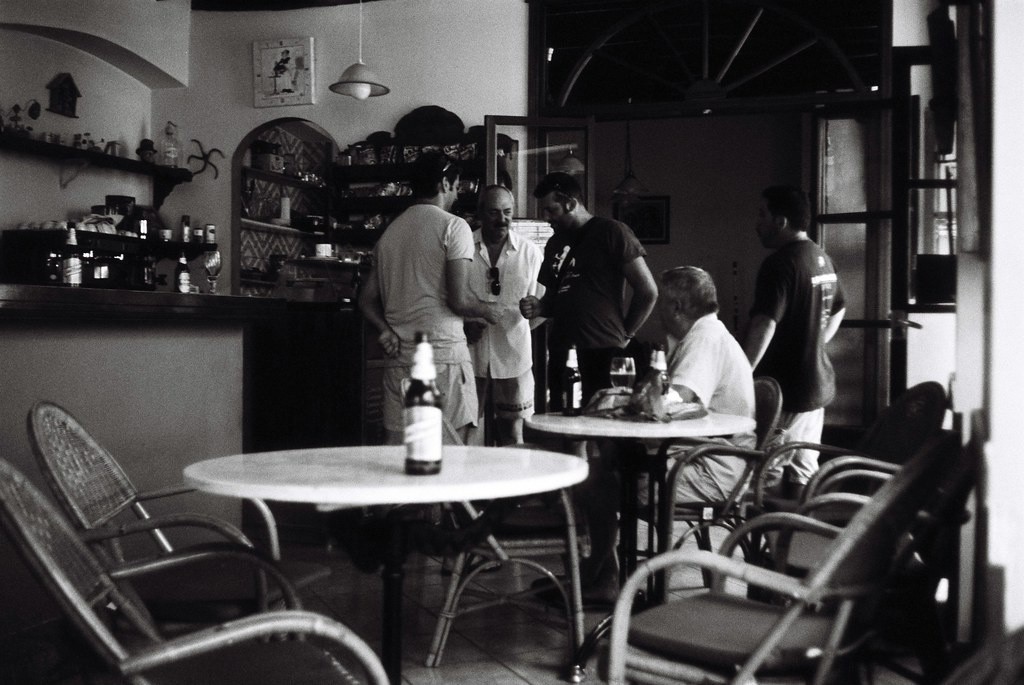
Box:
[159,229,173,241]
[316,243,332,258]
[609,356,636,388]
[15,219,96,232]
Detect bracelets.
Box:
[625,333,632,339]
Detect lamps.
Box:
[612,119,649,194]
[328,0,390,101]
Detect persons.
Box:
[519,172,659,407]
[359,163,508,576]
[744,185,846,498]
[464,185,548,448]
[533,265,757,610]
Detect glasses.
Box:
[489,267,501,296]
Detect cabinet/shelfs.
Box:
[331,105,515,275]
[0,136,218,264]
[241,164,330,288]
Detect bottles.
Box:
[648,343,669,392]
[60,223,83,288]
[180,215,216,243]
[161,125,179,166]
[562,344,583,416]
[174,250,190,293]
[401,329,443,475]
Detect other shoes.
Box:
[531,572,621,610]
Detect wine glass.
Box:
[203,250,223,295]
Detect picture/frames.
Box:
[252,37,315,107]
[613,195,670,245]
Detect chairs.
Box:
[0,373,974,685]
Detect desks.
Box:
[525,411,757,683]
[181,445,589,685]
